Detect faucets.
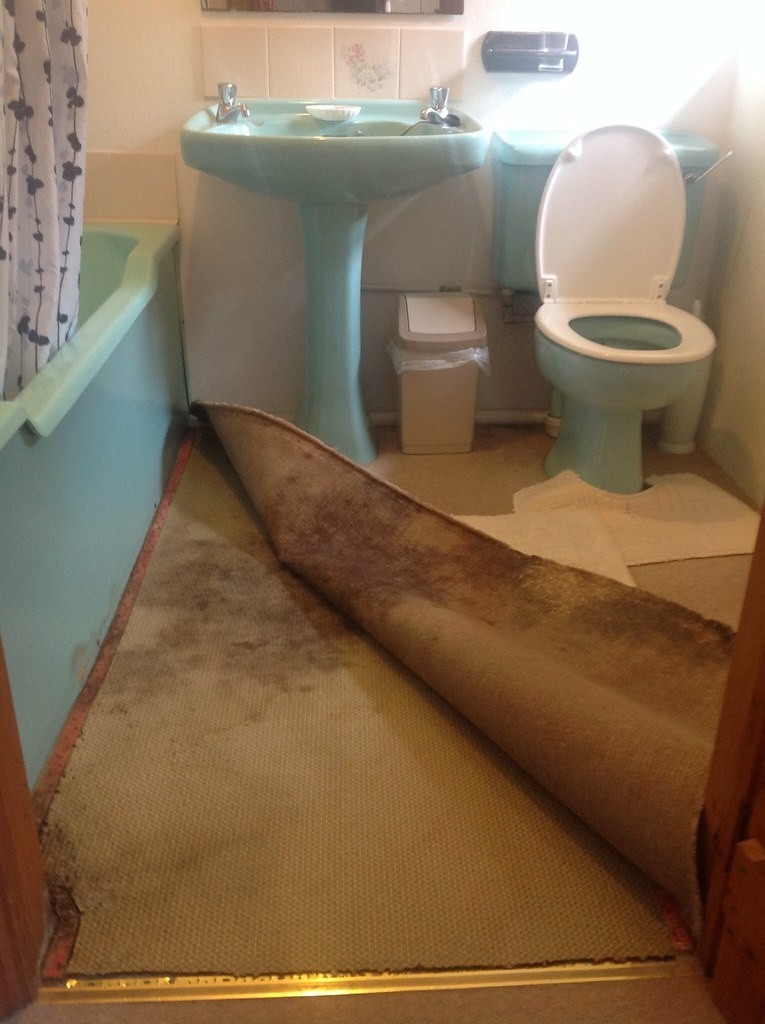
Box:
[420,106,447,125]
[217,103,251,122]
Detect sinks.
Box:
[178,97,492,204]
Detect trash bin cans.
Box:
[396,291,489,454]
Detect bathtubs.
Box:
[0,220,185,793]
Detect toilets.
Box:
[493,120,721,495]
